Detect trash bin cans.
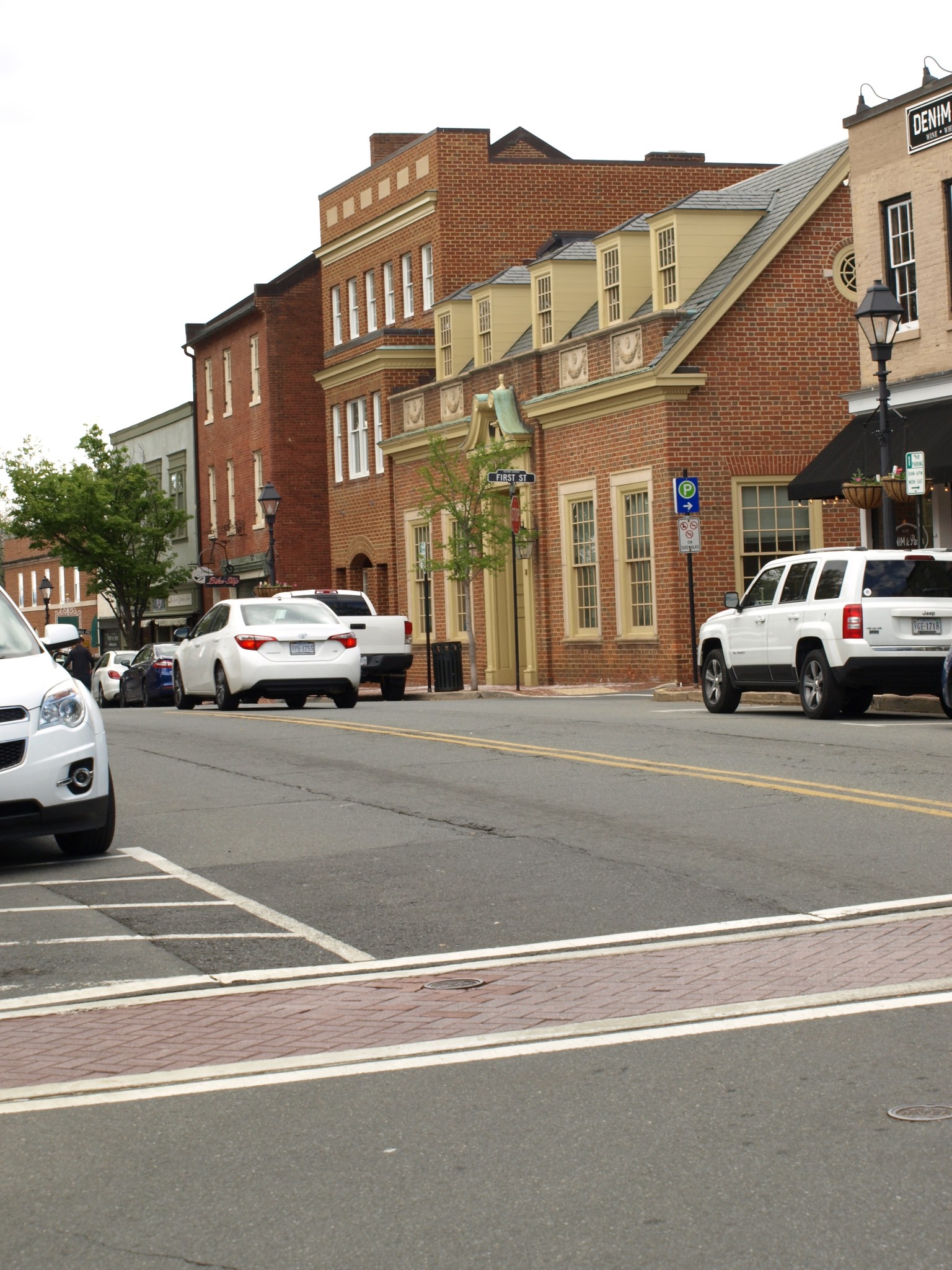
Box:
[431,641,464,692]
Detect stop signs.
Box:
[511,496,522,535]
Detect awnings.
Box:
[123,615,191,628]
[788,400,952,501]
[92,615,98,647]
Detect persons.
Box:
[64,637,94,690]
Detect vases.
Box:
[253,587,291,597]
[841,482,883,509]
[881,478,933,505]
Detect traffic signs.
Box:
[905,451,925,495]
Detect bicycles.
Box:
[198,536,235,574]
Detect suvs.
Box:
[693,547,952,722]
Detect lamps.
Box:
[798,500,802,507]
[458,541,479,557]
[921,56,952,89]
[855,83,890,115]
[515,509,539,560]
[944,482,948,491]
[822,498,826,504]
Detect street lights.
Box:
[38,576,54,624]
[257,481,283,587]
[855,278,900,549]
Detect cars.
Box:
[118,641,187,707]
[171,597,362,709]
[1,588,116,856]
[91,650,140,706]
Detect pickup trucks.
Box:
[275,590,415,699]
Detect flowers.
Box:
[851,468,883,485]
[259,580,297,588]
[888,468,906,479]
